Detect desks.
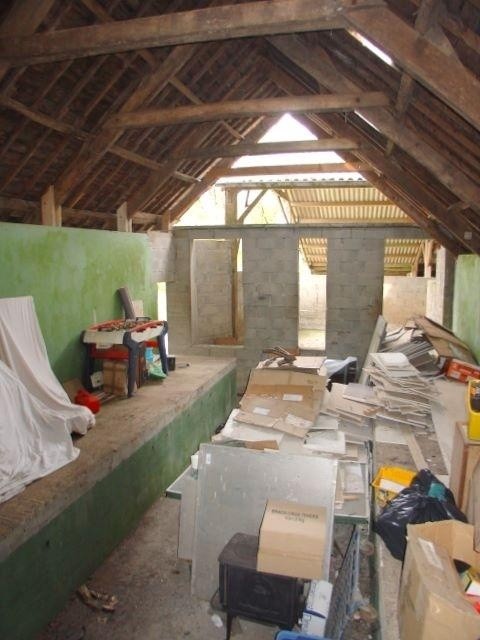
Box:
[80,319,169,399]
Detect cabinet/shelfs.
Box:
[218,532,306,639]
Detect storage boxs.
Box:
[396,421,480,640]
[256,498,327,579]
[443,358,480,384]
[101,353,146,397]
